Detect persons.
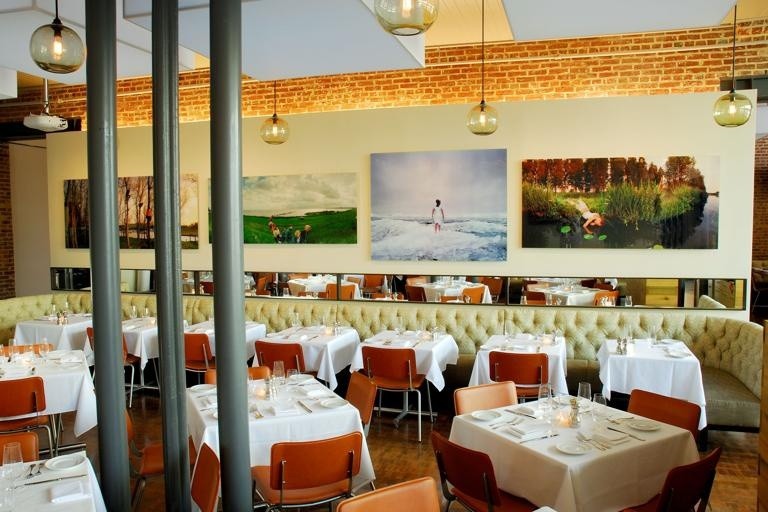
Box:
[264,217,315,243]
[569,194,606,233]
[430,199,446,233]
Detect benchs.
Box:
[505,305,738,428]
[2,291,336,358]
[337,300,504,382]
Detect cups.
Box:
[264,360,298,402]
[48,306,659,356]
[281,287,634,307]
[0,337,49,372]
[537,381,608,433]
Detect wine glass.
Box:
[0,442,24,512]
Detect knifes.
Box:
[24,473,88,486]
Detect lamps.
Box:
[29,1,87,73]
[373,0,440,38]
[461,0,501,136]
[712,3,753,130]
[256,81,290,146]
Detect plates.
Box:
[191,373,348,420]
[45,454,87,470]
[48,351,84,371]
[660,338,693,359]
[471,395,662,455]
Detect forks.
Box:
[27,460,45,479]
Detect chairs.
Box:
[488,351,549,402]
[430,380,724,512]
[183,365,378,511]
[0,312,98,512]
[248,273,620,307]
[85,316,365,409]
[360,346,426,444]
[336,475,439,511]
[125,411,199,490]
